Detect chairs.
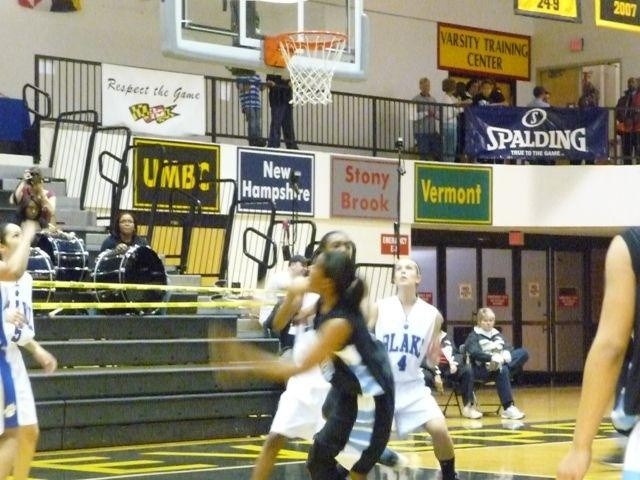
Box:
[437,325,507,417]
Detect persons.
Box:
[554,223,640,480]
[367,255,457,480]
[462,306,529,421]
[0,195,57,249]
[265,72,299,151]
[84,211,174,316]
[0,218,42,282]
[437,75,640,165]
[407,76,443,162]
[420,328,483,421]
[258,254,311,354]
[0,220,61,480]
[235,71,267,147]
[597,431,631,467]
[8,166,57,224]
[247,231,360,480]
[206,251,398,480]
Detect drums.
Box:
[90,243,167,316]
[36,231,90,295]
[25,247,58,316]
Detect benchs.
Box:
[0,165,287,455]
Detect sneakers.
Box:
[490,359,499,371]
[464,406,483,420]
[500,406,526,420]
